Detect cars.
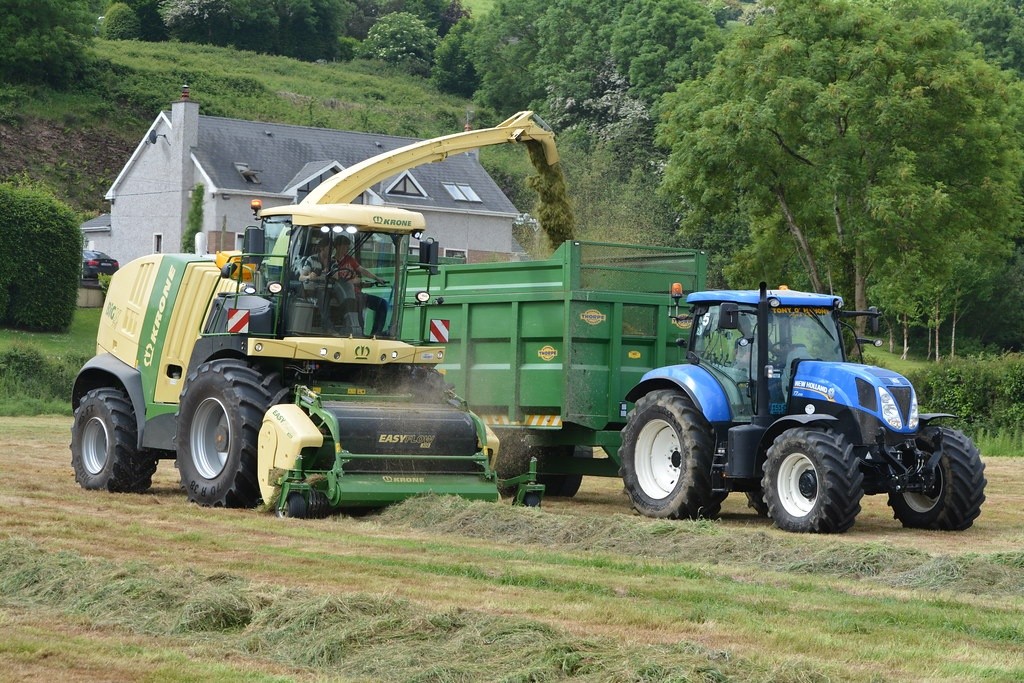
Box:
[80,251,120,279]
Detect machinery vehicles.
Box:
[255,233,987,537]
[69,111,561,520]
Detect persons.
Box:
[734,315,782,401]
[298,236,389,337]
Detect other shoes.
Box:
[371,332,385,339]
